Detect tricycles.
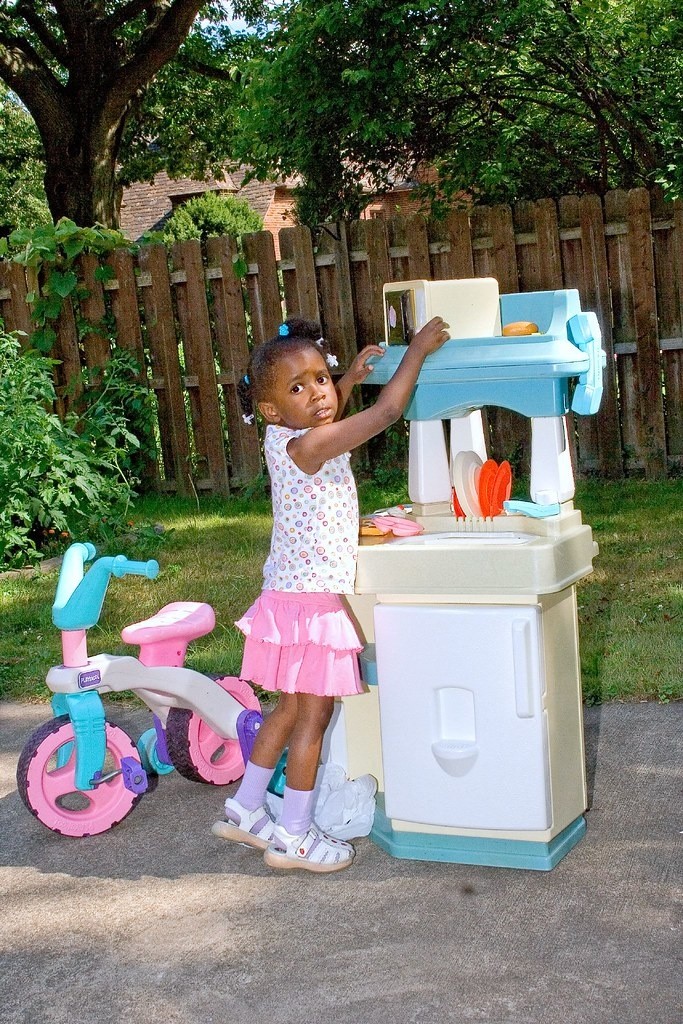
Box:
[17,542,290,839]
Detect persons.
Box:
[210,315,452,874]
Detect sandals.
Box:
[263,820,355,873]
[210,798,276,849]
